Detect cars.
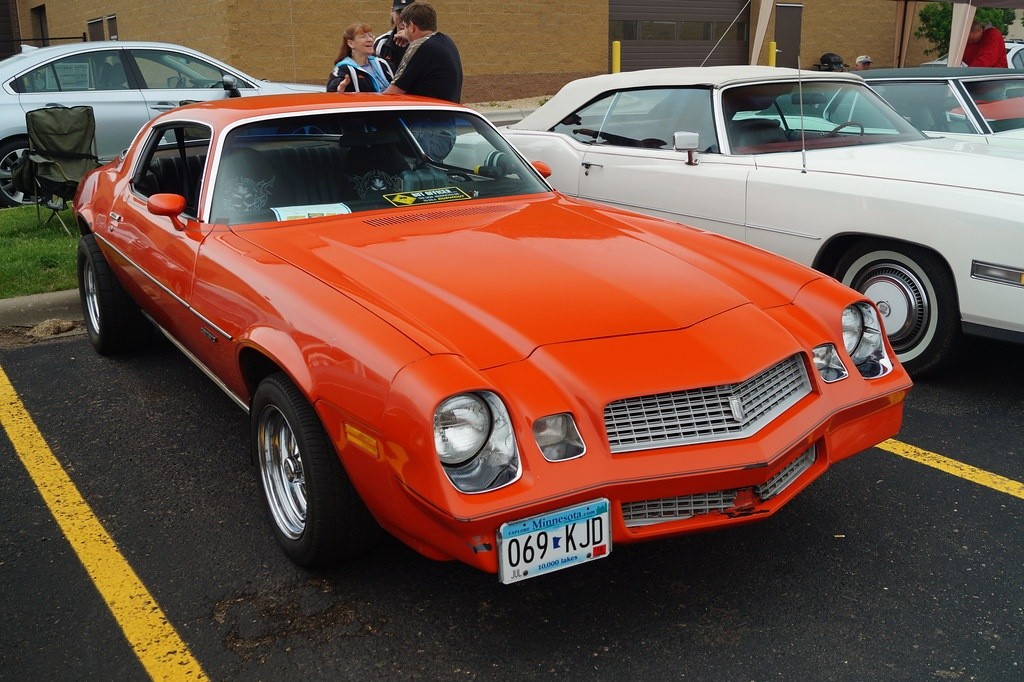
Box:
[0,40,328,208]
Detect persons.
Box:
[372,0,416,75]
[327,24,396,93]
[384,2,463,162]
[856,55,872,70]
[962,20,1008,98]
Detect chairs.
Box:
[226,147,285,210]
[109,63,127,89]
[733,126,786,146]
[341,144,412,201]
[98,62,113,89]
[10,105,104,237]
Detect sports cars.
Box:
[443,39,1024,385]
[73,92,914,586]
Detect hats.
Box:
[856,55,872,64]
[393,0,416,11]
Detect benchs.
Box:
[572,116,679,144]
[149,146,350,215]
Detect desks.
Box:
[813,63,850,72]
